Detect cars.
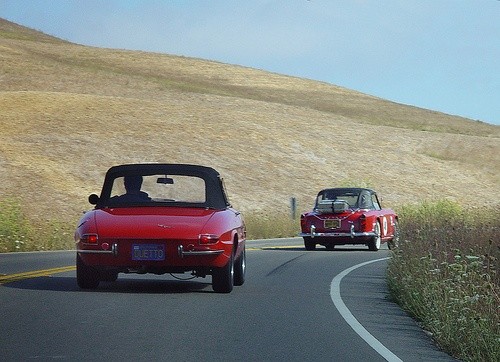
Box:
[298,187,399,251]
[74,162,246,293]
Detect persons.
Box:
[111,176,151,202]
[325,191,338,199]
